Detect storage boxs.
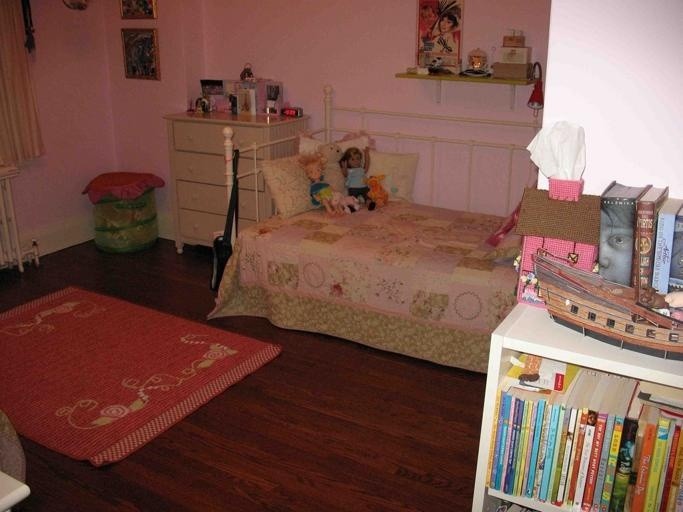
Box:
[499,44,531,64]
[491,61,534,80]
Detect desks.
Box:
[0,470,31,511]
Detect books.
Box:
[598,179,683,318]
[485,356,680,512]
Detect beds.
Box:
[216,83,541,375]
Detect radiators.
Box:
[0,164,40,276]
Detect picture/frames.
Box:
[119,27,161,81]
[119,0,158,20]
[236,88,255,116]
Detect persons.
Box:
[428,12,459,54]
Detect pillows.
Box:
[363,144,421,203]
[292,124,372,156]
[258,150,319,216]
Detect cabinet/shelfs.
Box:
[161,110,309,253]
[471,303,682,512]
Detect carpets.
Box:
[0,283,283,467]
